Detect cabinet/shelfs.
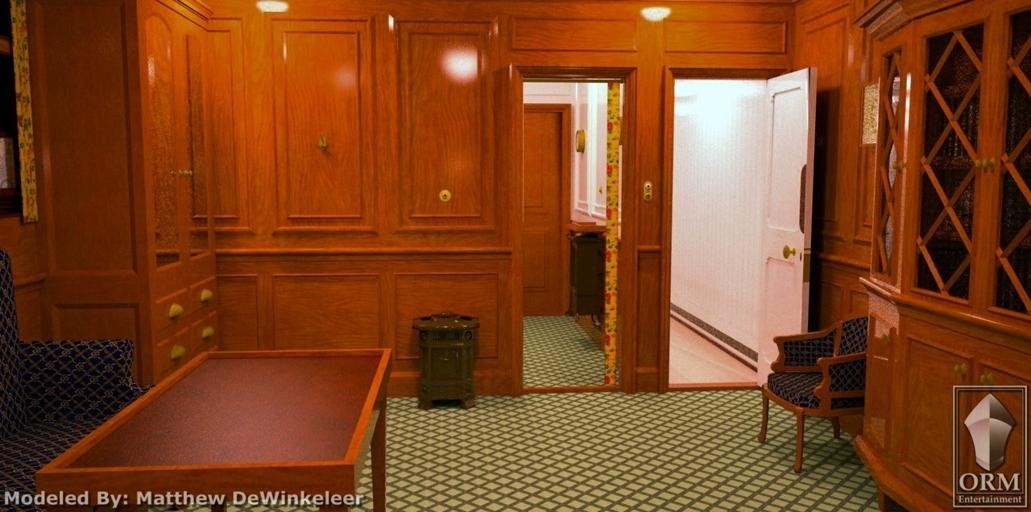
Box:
[7,0,523,401]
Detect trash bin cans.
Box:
[412,311,479,410]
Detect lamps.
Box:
[576,129,586,152]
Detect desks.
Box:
[34,346,392,512]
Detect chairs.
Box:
[0,248,163,512]
[758,310,872,462]
